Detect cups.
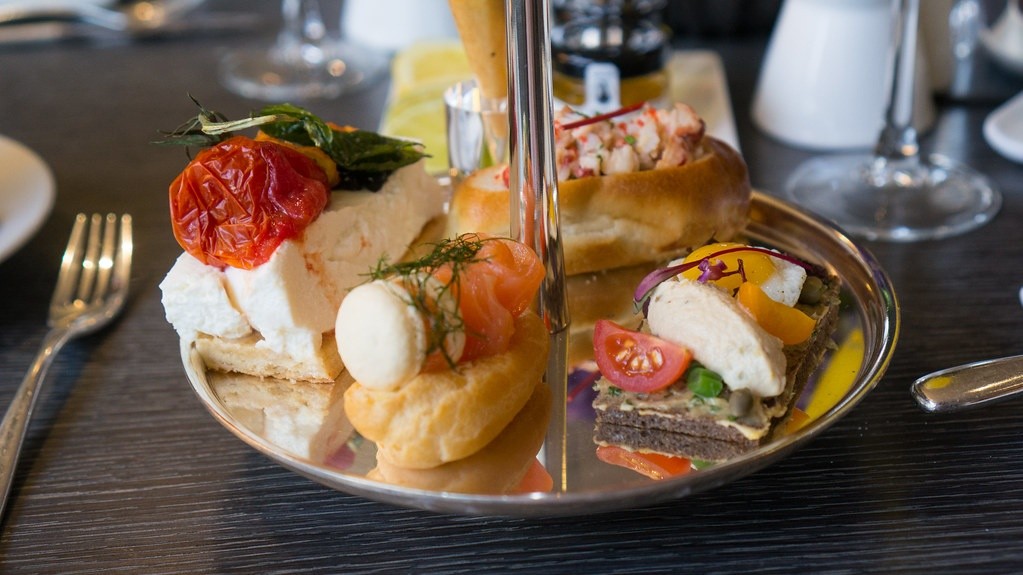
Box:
[443,77,510,175]
[548,0,675,116]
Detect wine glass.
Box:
[219,0,386,103]
[782,0,1004,240]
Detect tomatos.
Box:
[592,446,694,480]
[593,317,692,392]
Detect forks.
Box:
[0,213,133,517]
[1,1,266,39]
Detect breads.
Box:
[591,323,837,463]
[444,106,750,277]
[341,310,552,469]
[160,155,450,383]
[360,386,549,498]
[591,269,844,451]
[209,371,357,467]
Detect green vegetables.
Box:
[157,102,521,367]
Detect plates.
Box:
[553,48,741,154]
[1,136,56,265]
